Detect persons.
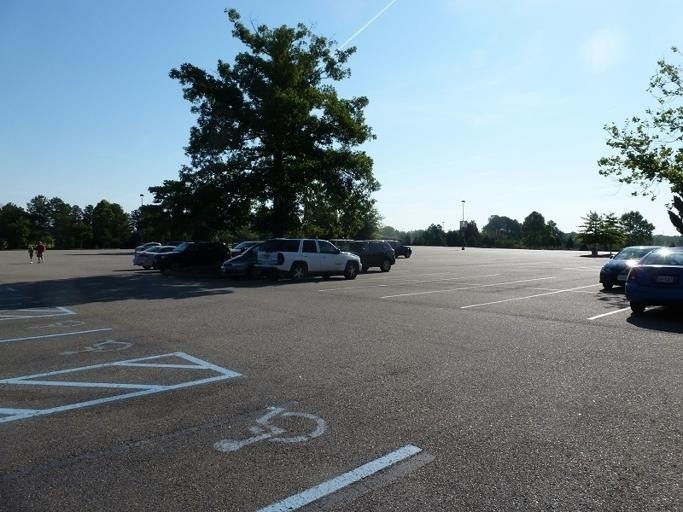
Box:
[28,241,46,264]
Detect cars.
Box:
[623,246,682,313]
[598,246,665,288]
[134,241,266,279]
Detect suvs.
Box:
[254,238,362,281]
[328,237,412,272]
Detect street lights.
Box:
[139,192,144,207]
[461,199,466,250]
